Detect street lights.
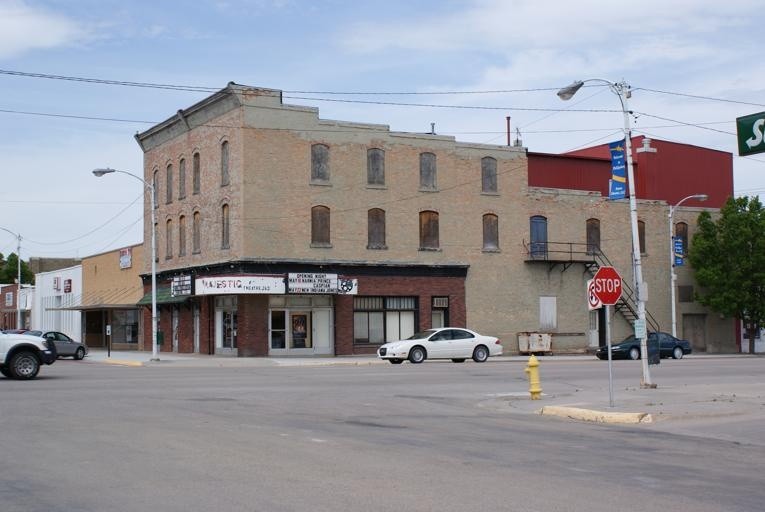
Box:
[556,77,658,389]
[91,168,157,357]
[668,193,707,337]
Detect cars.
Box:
[376,327,504,363]
[0,329,88,380]
[596,332,689,359]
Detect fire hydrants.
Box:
[525,354,542,400]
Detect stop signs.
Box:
[592,266,622,306]
